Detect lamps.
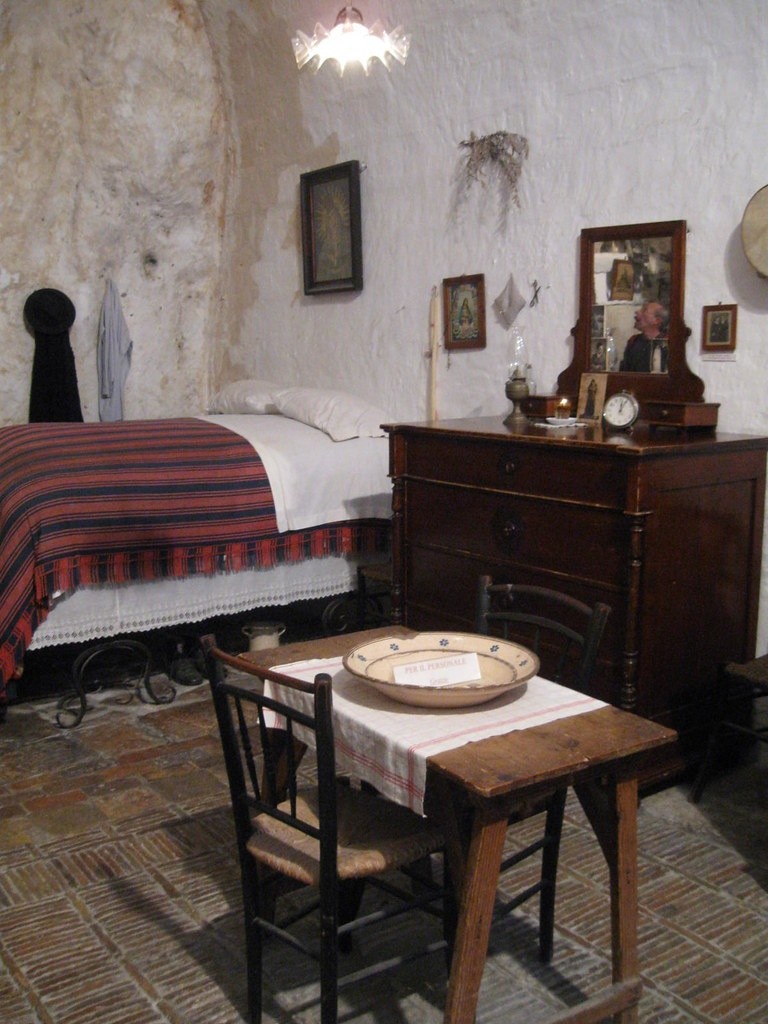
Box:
[503,323,530,424]
[290,1,411,78]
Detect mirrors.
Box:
[557,219,712,401]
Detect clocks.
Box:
[602,389,639,433]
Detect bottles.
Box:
[524,364,536,396]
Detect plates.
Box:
[534,423,588,431]
[342,631,540,709]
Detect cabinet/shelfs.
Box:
[590,301,645,371]
[379,411,768,799]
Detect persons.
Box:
[584,379,598,416]
[620,300,670,374]
[591,342,606,368]
[710,314,730,342]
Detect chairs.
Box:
[200,632,468,1024]
[414,574,614,962]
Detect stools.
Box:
[359,561,393,628]
[687,654,767,804]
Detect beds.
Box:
[0,412,394,724]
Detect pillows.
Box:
[273,387,397,442]
[203,379,295,414]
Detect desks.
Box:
[236,623,682,1024]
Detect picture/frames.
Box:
[609,258,636,301]
[299,159,365,295]
[701,300,737,352]
[442,273,486,350]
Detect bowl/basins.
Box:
[546,417,578,426]
